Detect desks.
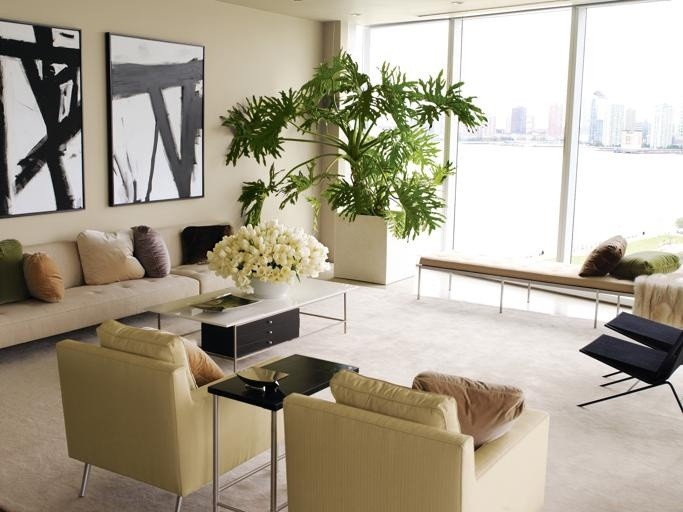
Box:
[205,353,358,510]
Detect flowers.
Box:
[204,220,340,294]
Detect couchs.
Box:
[129,220,242,331]
[50,339,285,511]
[0,231,202,353]
[280,388,549,511]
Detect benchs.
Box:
[415,248,635,329]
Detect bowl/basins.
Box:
[237,367,289,388]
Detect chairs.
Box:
[574,331,681,414]
[600,308,682,389]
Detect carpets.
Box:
[3,276,680,510]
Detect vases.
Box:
[242,264,300,301]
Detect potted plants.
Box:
[219,45,492,288]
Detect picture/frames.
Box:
[102,31,205,207]
[0,17,85,219]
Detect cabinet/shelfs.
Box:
[196,306,300,362]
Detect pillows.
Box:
[328,368,461,438]
[21,250,64,304]
[409,370,528,448]
[140,324,224,387]
[577,234,628,279]
[608,249,681,280]
[178,224,233,267]
[0,238,29,306]
[75,229,146,286]
[129,224,171,278]
[95,317,192,369]
